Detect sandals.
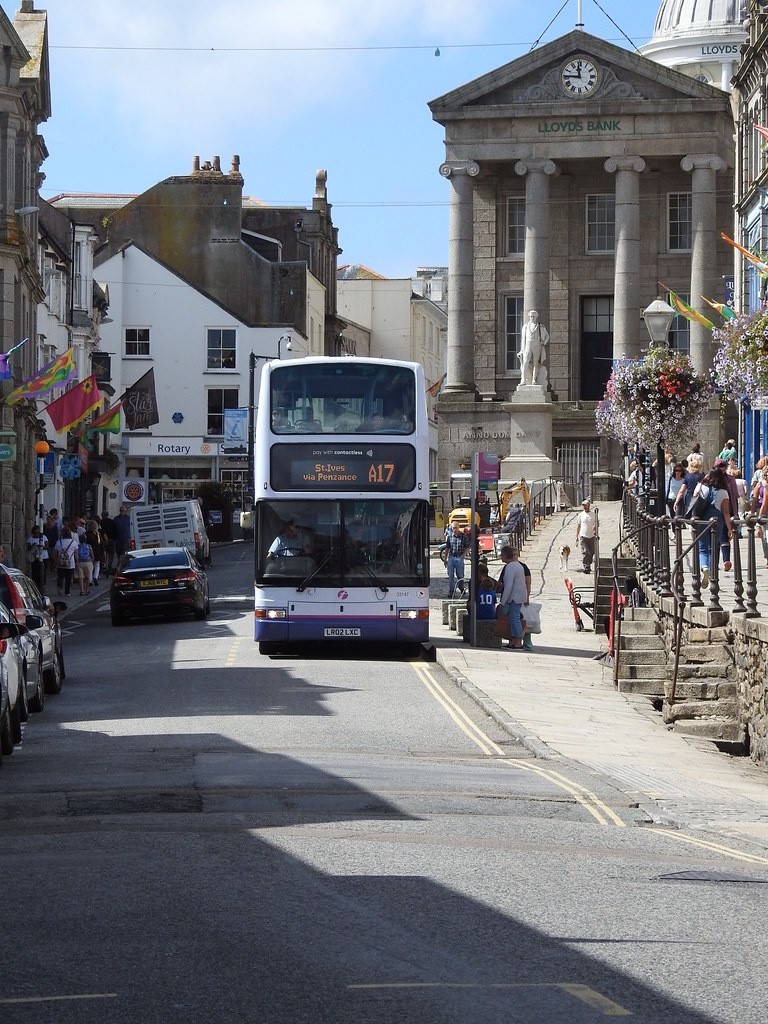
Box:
[80,592,90,596]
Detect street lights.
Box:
[34,440,50,591]
[643,296,677,567]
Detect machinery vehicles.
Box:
[449,477,534,532]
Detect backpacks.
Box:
[77,543,89,562]
[56,538,75,569]
[688,456,701,474]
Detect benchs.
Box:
[569,586,595,617]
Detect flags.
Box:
[0,339,28,382]
[699,295,737,323]
[721,231,768,281]
[655,281,714,333]
[44,375,104,436]
[119,367,162,430]
[427,370,448,398]
[75,399,126,450]
[1,345,78,407]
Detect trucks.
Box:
[129,499,210,568]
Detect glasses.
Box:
[675,469,681,472]
[120,510,125,512]
[513,553,517,557]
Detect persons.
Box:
[304,407,321,432]
[272,408,284,427]
[516,310,549,386]
[711,459,739,571]
[500,546,528,650]
[444,522,467,597]
[477,578,496,619]
[1,628,11,638]
[27,525,48,588]
[370,412,385,430]
[686,470,734,589]
[85,521,102,586]
[497,547,533,651]
[621,439,747,539]
[74,535,93,596]
[54,528,78,597]
[397,415,410,425]
[575,500,597,573]
[43,506,131,572]
[476,560,499,593]
[267,524,312,559]
[503,504,523,533]
[751,457,768,568]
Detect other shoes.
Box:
[66,593,71,597]
[89,583,92,587]
[583,569,590,574]
[448,594,455,599]
[700,568,710,590]
[93,578,99,587]
[57,587,62,595]
[501,645,523,650]
[461,590,465,594]
[724,561,732,571]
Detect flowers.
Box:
[595,300,768,456]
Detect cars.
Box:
[1,563,68,755]
[108,546,210,626]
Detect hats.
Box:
[714,460,728,467]
[582,500,590,506]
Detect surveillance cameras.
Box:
[287,342,293,351]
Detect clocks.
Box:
[564,56,598,93]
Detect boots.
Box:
[521,633,534,651]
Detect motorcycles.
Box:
[438,525,480,562]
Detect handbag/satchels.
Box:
[493,604,526,641]
[684,484,708,519]
[94,532,103,562]
[519,603,542,634]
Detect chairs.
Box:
[460,497,471,506]
[273,419,321,433]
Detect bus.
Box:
[251,355,432,654]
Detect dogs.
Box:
[557,544,570,572]
